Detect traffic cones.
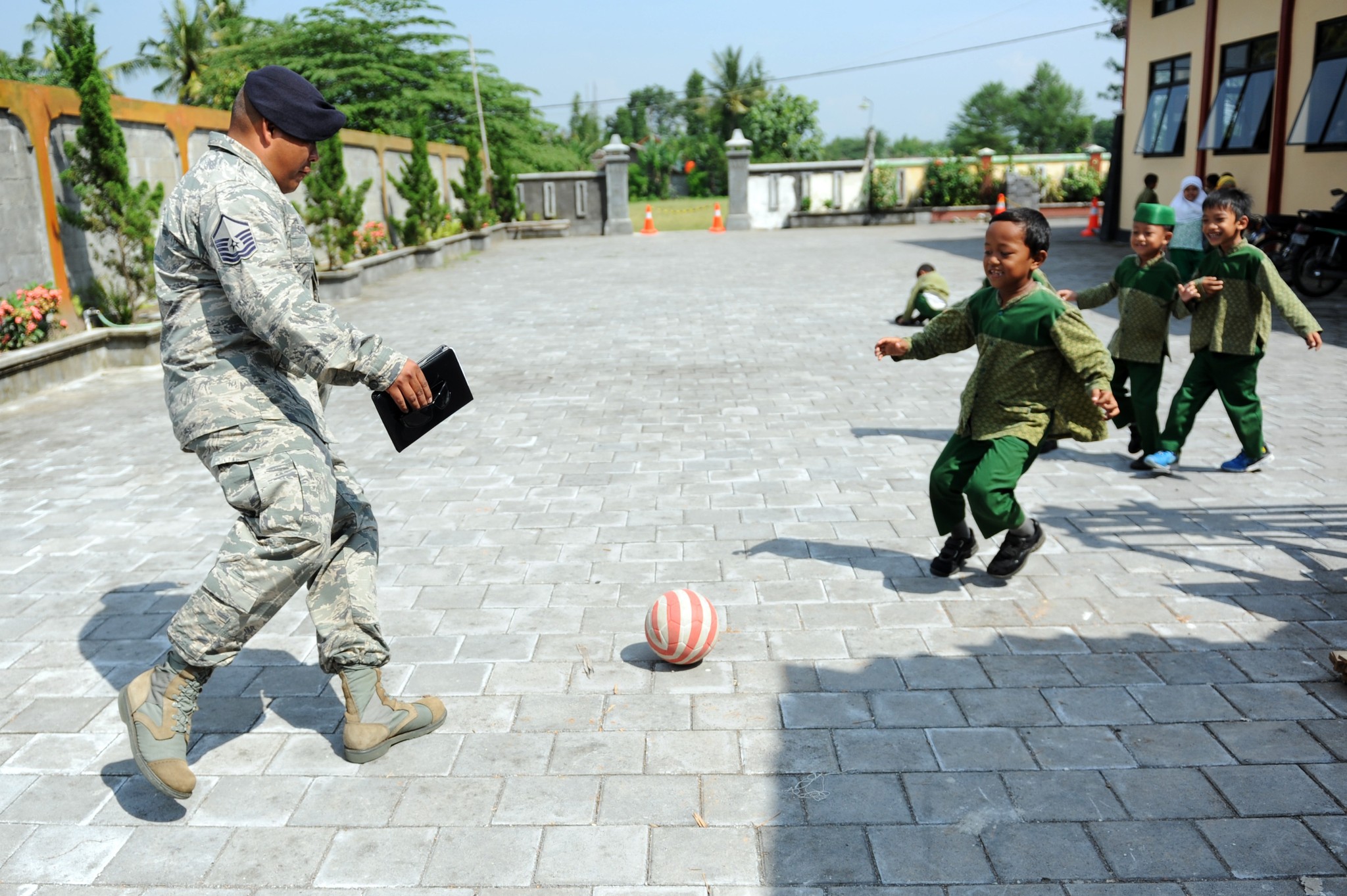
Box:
[1081,196,1102,236]
[710,202,726,231]
[640,204,658,233]
[994,193,1007,215]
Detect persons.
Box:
[1167,171,1235,281]
[874,208,1120,578]
[118,67,449,800]
[1056,202,1201,471]
[1135,173,1159,210]
[1144,189,1322,473]
[899,264,950,326]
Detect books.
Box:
[372,344,474,453]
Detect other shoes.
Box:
[1040,439,1057,453]
[1128,426,1142,453]
[1131,451,1157,471]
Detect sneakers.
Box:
[1221,446,1275,473]
[986,517,1044,579]
[1144,449,1180,471]
[929,527,978,575]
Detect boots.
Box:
[117,644,213,799]
[334,662,449,763]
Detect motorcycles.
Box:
[1246,186,1347,296]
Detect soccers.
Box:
[643,590,721,665]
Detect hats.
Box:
[244,64,347,143]
[1134,204,1176,225]
